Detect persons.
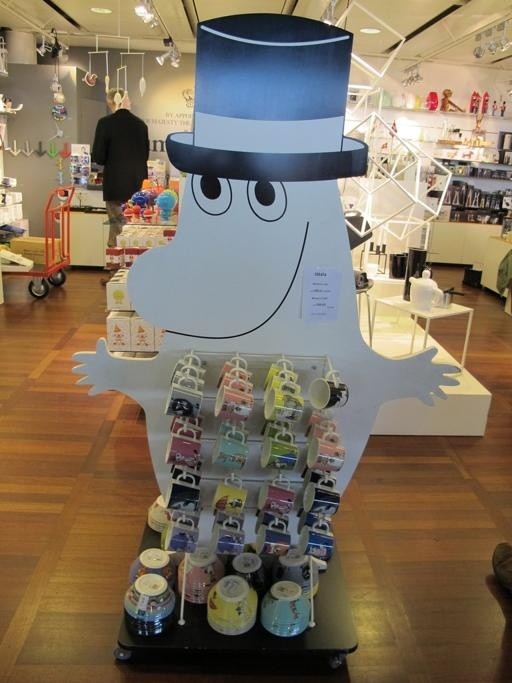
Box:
[91,87,150,287]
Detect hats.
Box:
[165,13,369,183]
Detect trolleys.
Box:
[0,186,75,300]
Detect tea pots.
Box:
[408,270,443,312]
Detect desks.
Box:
[103,209,178,225]
[371,294,474,372]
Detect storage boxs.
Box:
[10,236,63,264]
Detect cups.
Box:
[164,353,348,560]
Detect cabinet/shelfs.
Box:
[424,220,505,271]
[480,229,511,298]
[60,184,110,267]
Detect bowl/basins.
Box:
[125,546,321,637]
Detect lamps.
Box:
[156,36,181,68]
[135,0,159,28]
